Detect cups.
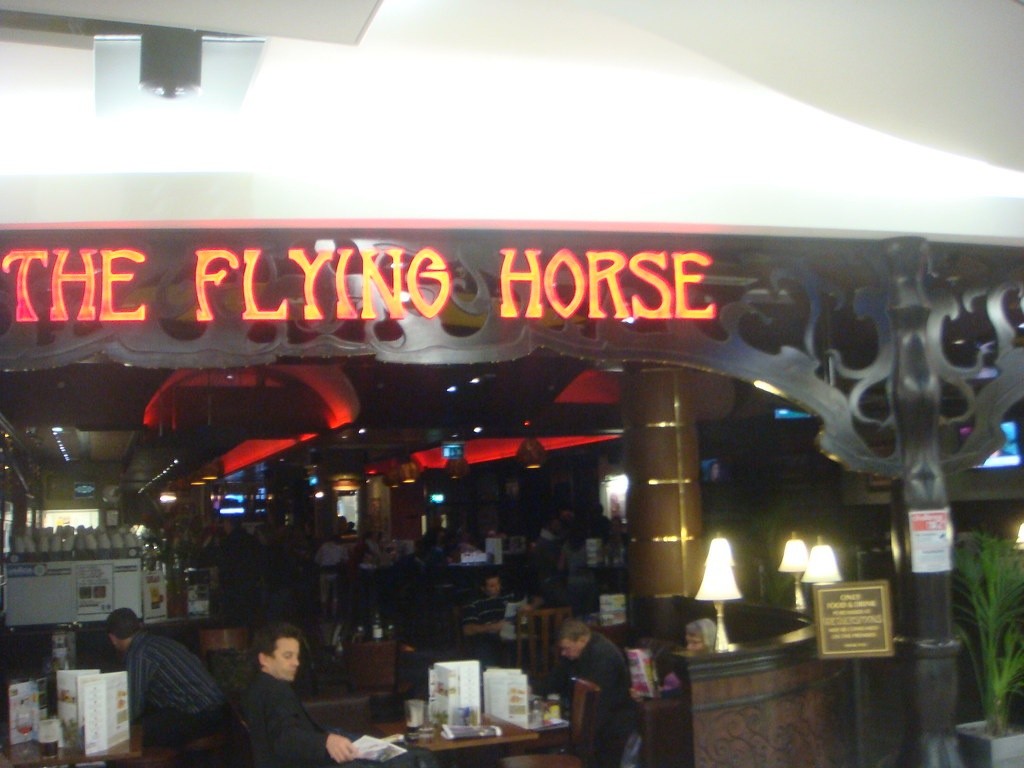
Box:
[39,720,58,759]
[416,706,437,742]
[12,526,140,553]
[405,699,425,740]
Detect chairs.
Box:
[199,607,720,768]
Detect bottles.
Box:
[372,612,383,641]
[547,694,562,724]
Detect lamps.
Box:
[515,437,546,468]
[446,459,470,478]
[191,461,219,484]
[385,459,419,488]
[693,533,744,652]
[778,532,809,610]
[800,536,843,583]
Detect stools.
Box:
[123,726,227,768]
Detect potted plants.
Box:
[950,532,1024,768]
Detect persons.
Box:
[247,623,441,768]
[105,515,637,746]
[620,618,718,768]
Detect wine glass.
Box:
[15,712,36,759]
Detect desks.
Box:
[0,724,141,768]
[582,609,628,646]
[375,711,539,768]
